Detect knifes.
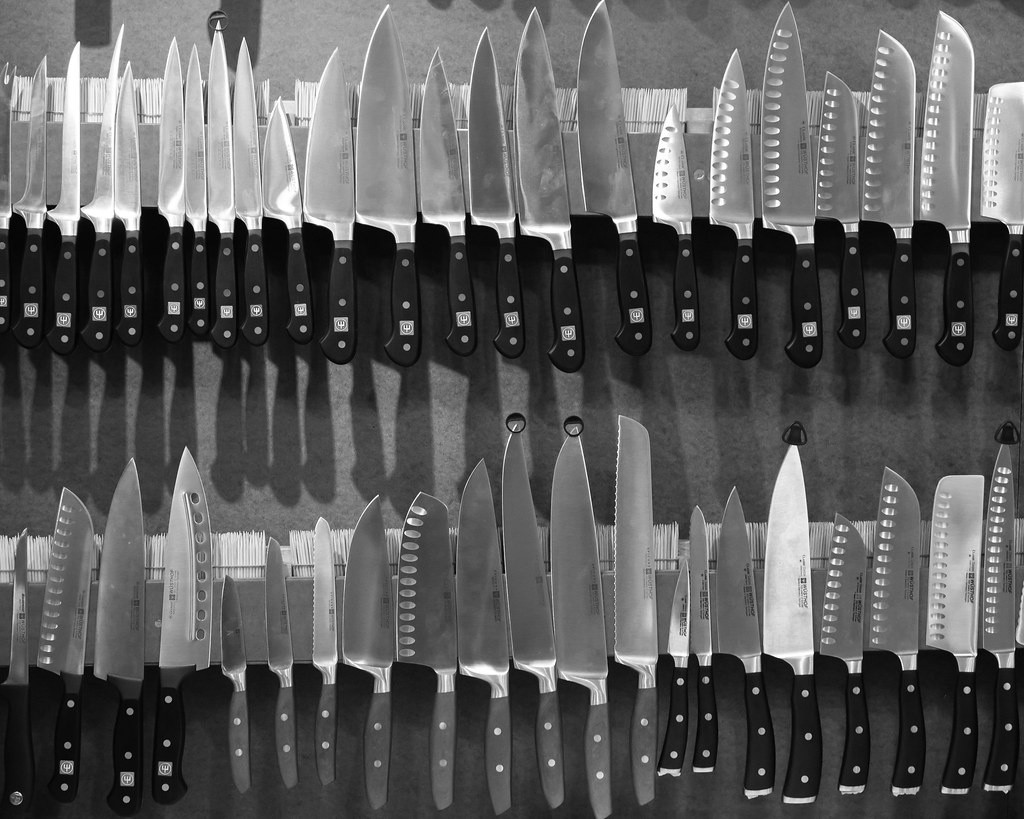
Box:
[2,526,35,819]
[260,94,316,347]
[498,422,567,811]
[264,536,300,791]
[355,3,587,375]
[90,455,148,816]
[655,0,1024,371]
[311,455,514,818]
[147,442,216,806]
[574,0,654,362]
[0,18,272,352]
[301,44,356,365]
[34,485,98,805]
[548,409,1024,819]
[217,573,255,795]
